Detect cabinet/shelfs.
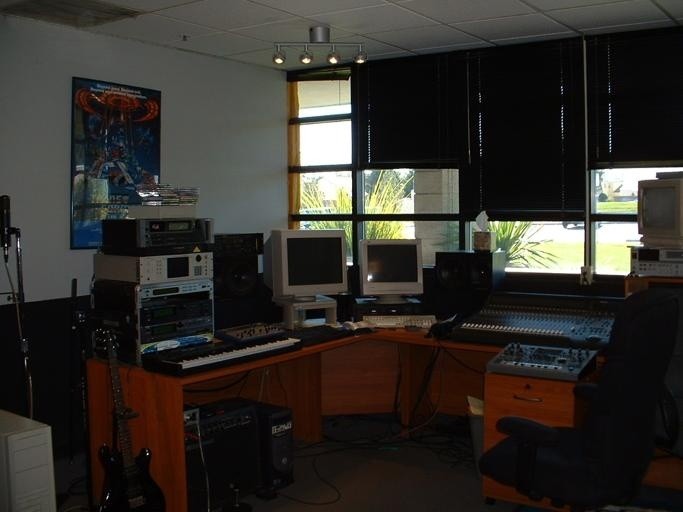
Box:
[480,373,573,509]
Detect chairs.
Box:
[481,286,681,512]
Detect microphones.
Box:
[0,195,10,247]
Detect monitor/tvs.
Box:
[638,178,683,247]
[263,230,348,301]
[358,239,424,304]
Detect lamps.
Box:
[272,25,367,70]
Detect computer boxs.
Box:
[254,409,294,488]
[354,305,419,321]
[631,248,683,277]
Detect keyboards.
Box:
[363,315,436,329]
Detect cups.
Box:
[579,264,594,287]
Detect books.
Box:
[132,182,198,207]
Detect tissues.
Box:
[471,210,498,253]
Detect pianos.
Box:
[141,334,304,375]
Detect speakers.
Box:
[214,233,264,297]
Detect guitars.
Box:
[96,325,167,512]
